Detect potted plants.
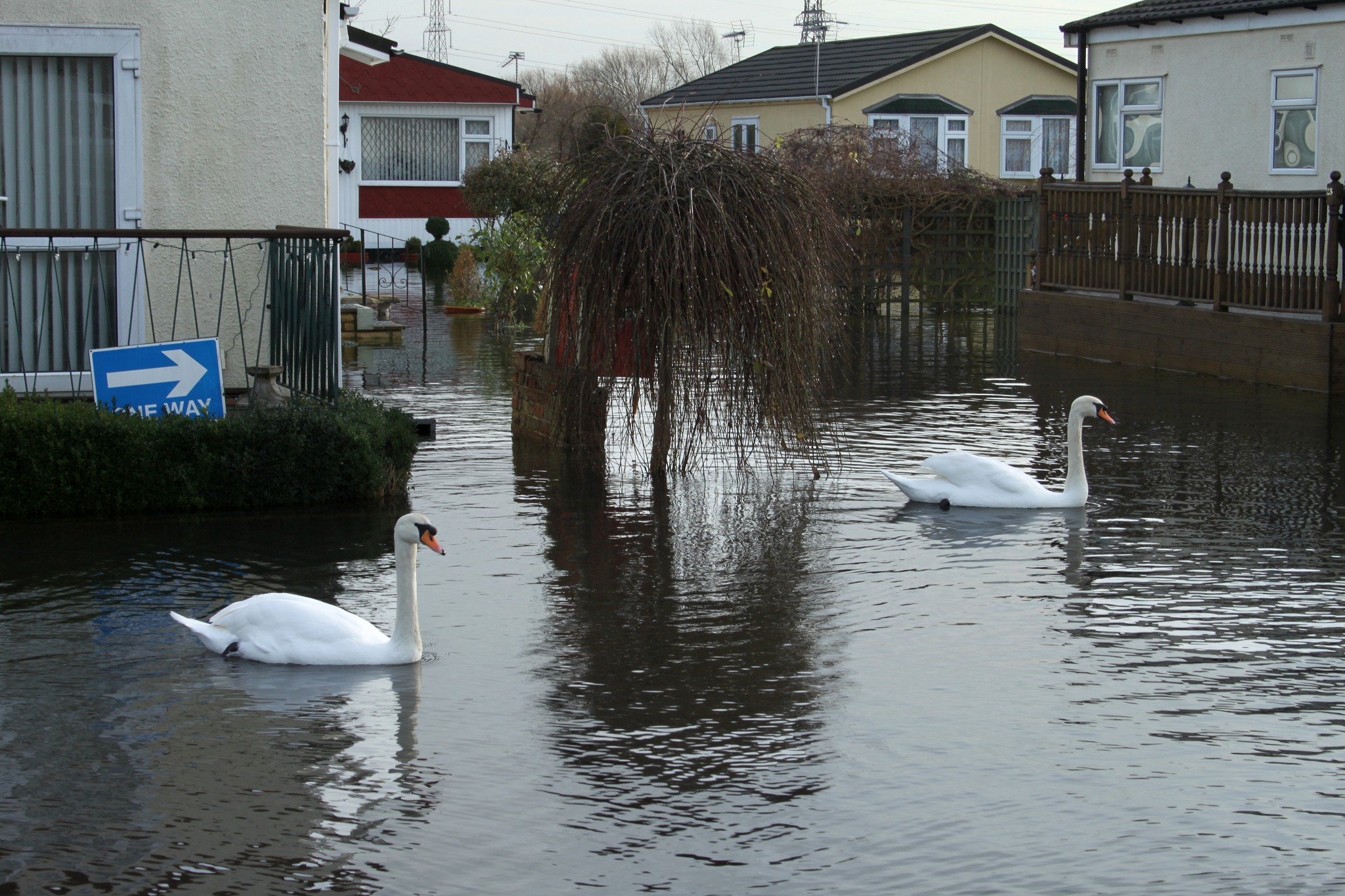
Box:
[338,236,369,265]
[443,245,487,315]
[401,237,422,267]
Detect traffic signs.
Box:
[88,338,227,425]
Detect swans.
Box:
[878,395,1116,508]
[168,513,446,665]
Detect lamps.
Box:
[340,112,350,135]
